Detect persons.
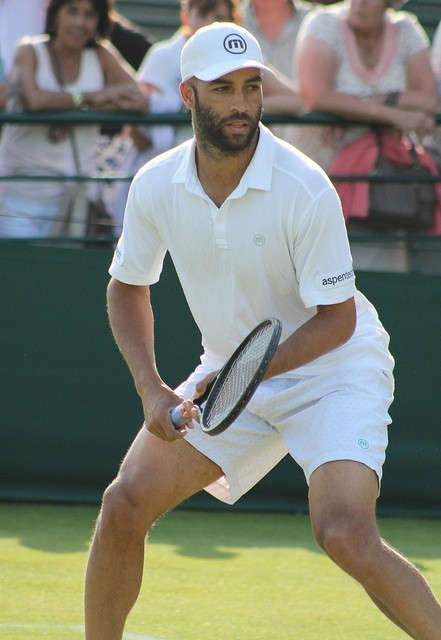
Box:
[298,1,436,272]
[107,0,304,250]
[1,0,147,241]
[69,1,153,238]
[87,20,441,640]
[236,0,312,78]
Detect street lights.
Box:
[367,159,435,230]
[52,183,113,248]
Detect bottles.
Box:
[179,21,276,82]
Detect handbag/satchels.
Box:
[68,88,87,116]
